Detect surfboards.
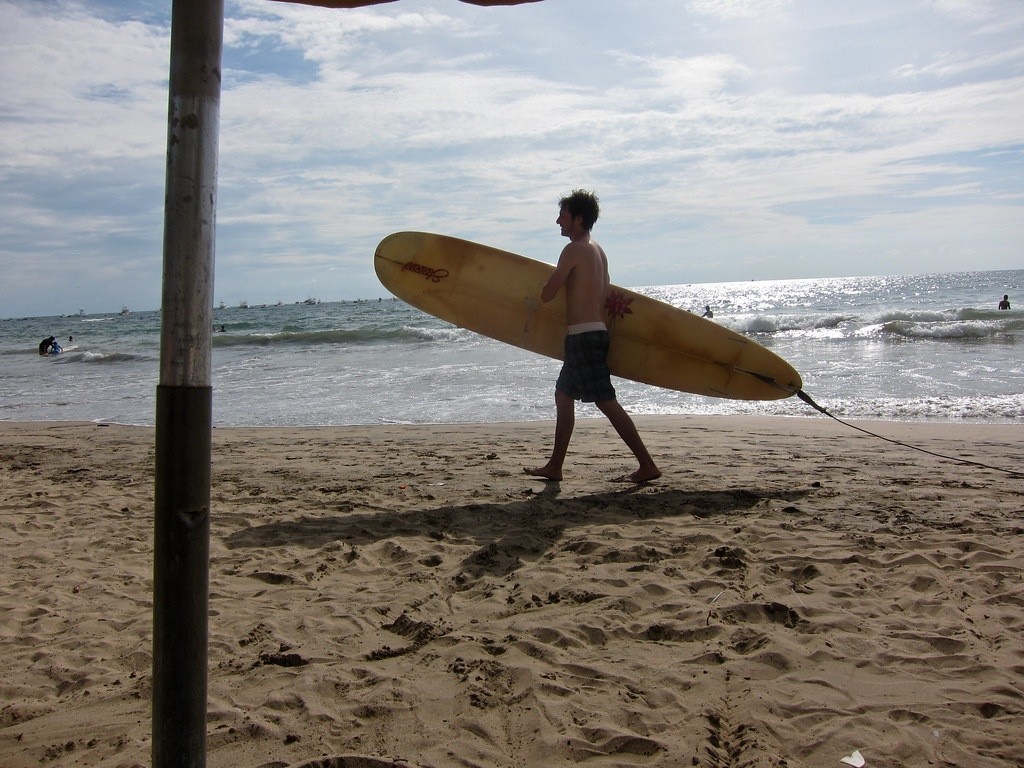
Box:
[371,228,803,402]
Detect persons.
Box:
[221,326,226,332]
[69,336,72,341]
[523,190,662,482]
[701,306,713,318]
[39,336,63,355]
[999,295,1010,310]
